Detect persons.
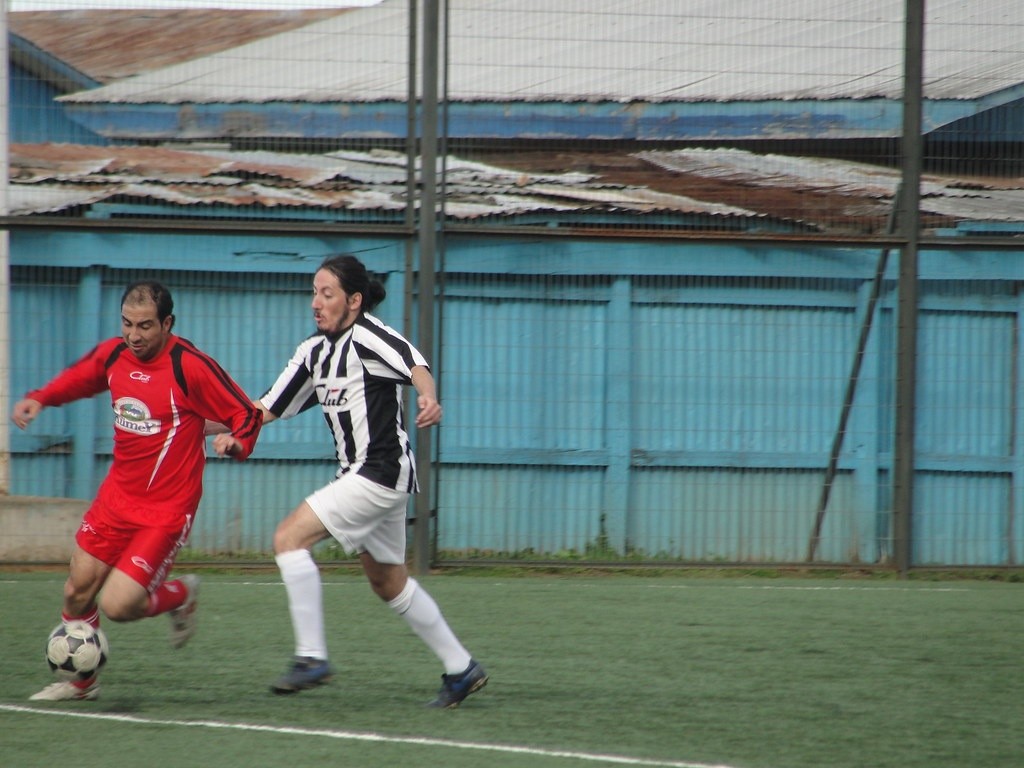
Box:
[11,281,263,702]
[204,256,489,709]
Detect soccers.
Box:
[45,620,110,684]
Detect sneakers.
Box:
[27,678,101,701]
[424,658,489,709]
[268,655,335,696]
[167,573,200,648]
